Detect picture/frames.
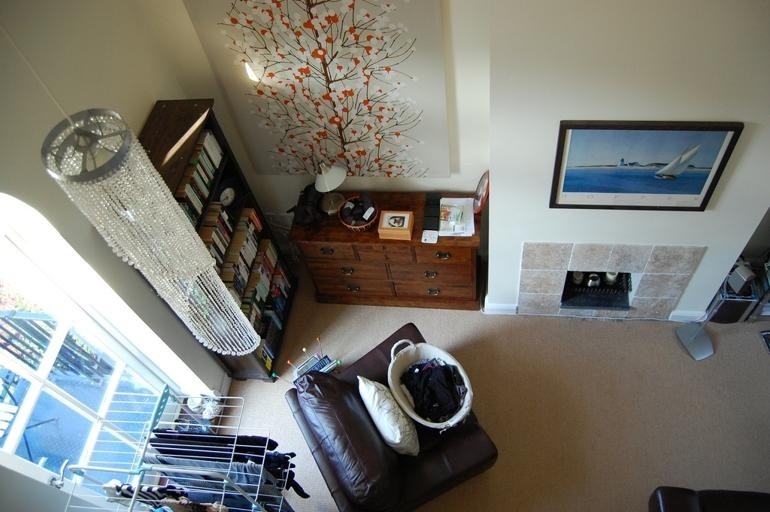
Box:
[548,119,745,212]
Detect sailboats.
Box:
[653,143,702,180]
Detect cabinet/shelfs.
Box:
[100,98,303,383]
[285,188,483,312]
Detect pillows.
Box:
[355,375,420,456]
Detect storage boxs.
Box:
[378,210,415,240]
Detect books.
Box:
[173,131,292,373]
[439,198,476,237]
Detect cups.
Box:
[586,273,601,287]
[605,271,619,283]
[572,273,584,285]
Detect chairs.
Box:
[285,322,498,511]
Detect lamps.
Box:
[1,24,261,356]
[314,160,349,215]
